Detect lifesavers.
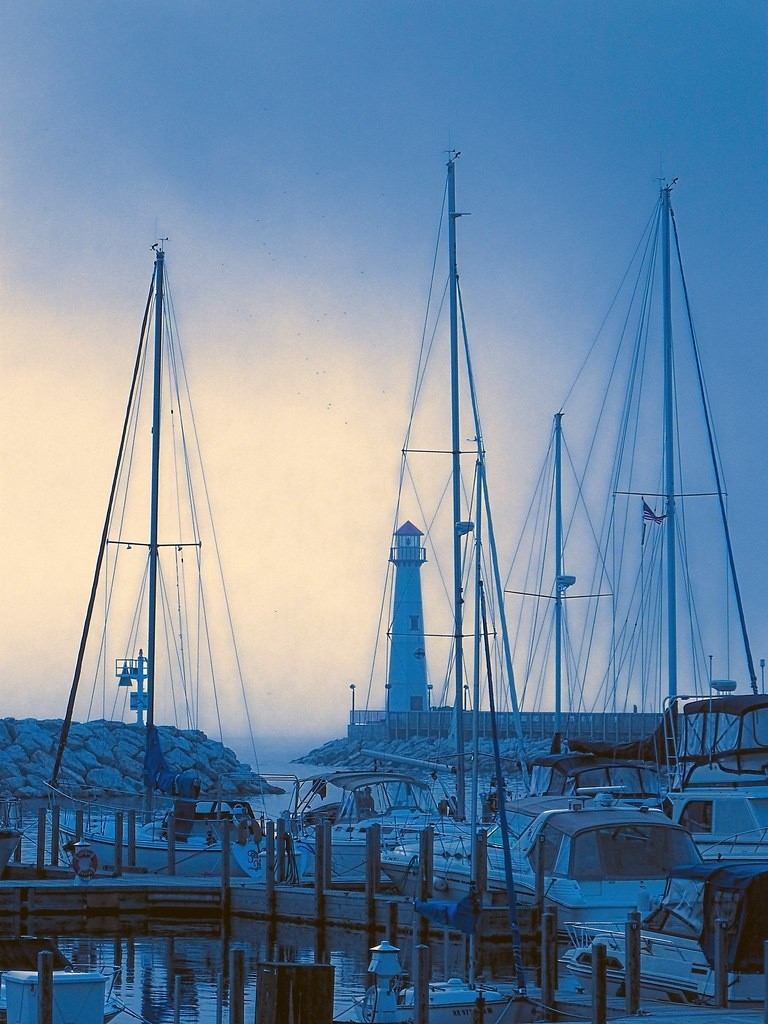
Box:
[73,849,97,876]
[239,819,262,844]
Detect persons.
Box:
[362,787,378,815]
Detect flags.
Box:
[643,500,668,525]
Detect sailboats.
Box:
[0,151,768,1024]
